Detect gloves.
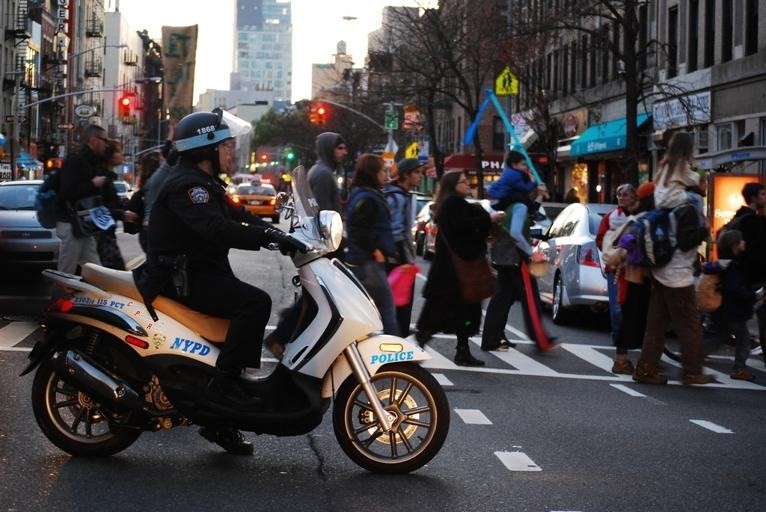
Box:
[265,228,306,258]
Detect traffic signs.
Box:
[385,110,399,129]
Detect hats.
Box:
[635,183,655,199]
[692,167,705,179]
[397,158,427,175]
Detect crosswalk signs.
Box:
[495,64,519,97]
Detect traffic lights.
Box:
[261,154,268,160]
[119,97,130,116]
[317,107,326,115]
[287,152,294,159]
[118,97,131,107]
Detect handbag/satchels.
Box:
[73,206,116,239]
[451,255,497,300]
[638,208,675,268]
[602,215,641,267]
[492,239,522,266]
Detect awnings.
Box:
[570,114,650,157]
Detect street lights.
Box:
[108,75,162,140]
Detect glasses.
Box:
[97,136,109,144]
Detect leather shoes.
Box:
[205,368,263,411]
[197,427,253,455]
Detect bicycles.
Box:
[664,252,765,363]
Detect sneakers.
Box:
[613,361,755,383]
[454,334,563,366]
[529,207,546,221]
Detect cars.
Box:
[230,173,281,223]
[411,195,492,261]
[112,181,135,200]
[1,178,61,276]
[518,201,618,324]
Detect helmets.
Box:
[172,107,252,152]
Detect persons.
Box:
[564,188,580,204]
[595,132,765,384]
[138,110,308,455]
[308,132,424,338]
[416,150,562,368]
[53,124,137,275]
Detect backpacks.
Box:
[694,272,722,312]
[35,169,64,228]
[123,188,147,234]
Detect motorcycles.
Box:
[18,166,459,475]
